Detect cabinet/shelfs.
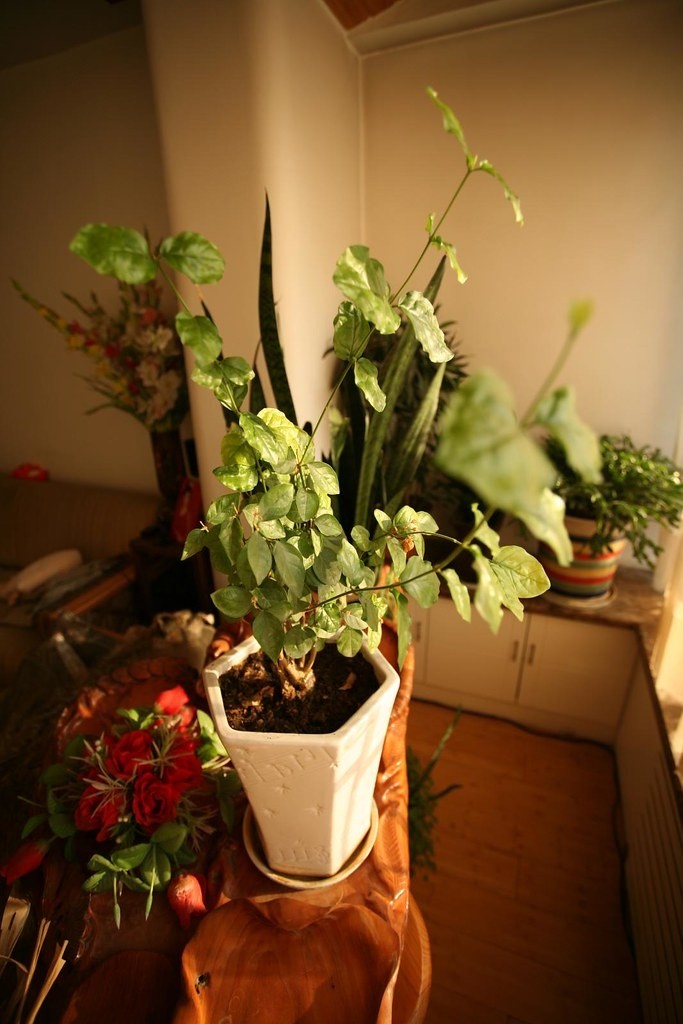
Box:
[402,593,638,743]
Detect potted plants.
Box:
[68,88,603,877]
[508,431,683,597]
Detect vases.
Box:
[149,426,191,510]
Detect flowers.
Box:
[9,227,190,434]
[2,687,243,930]
